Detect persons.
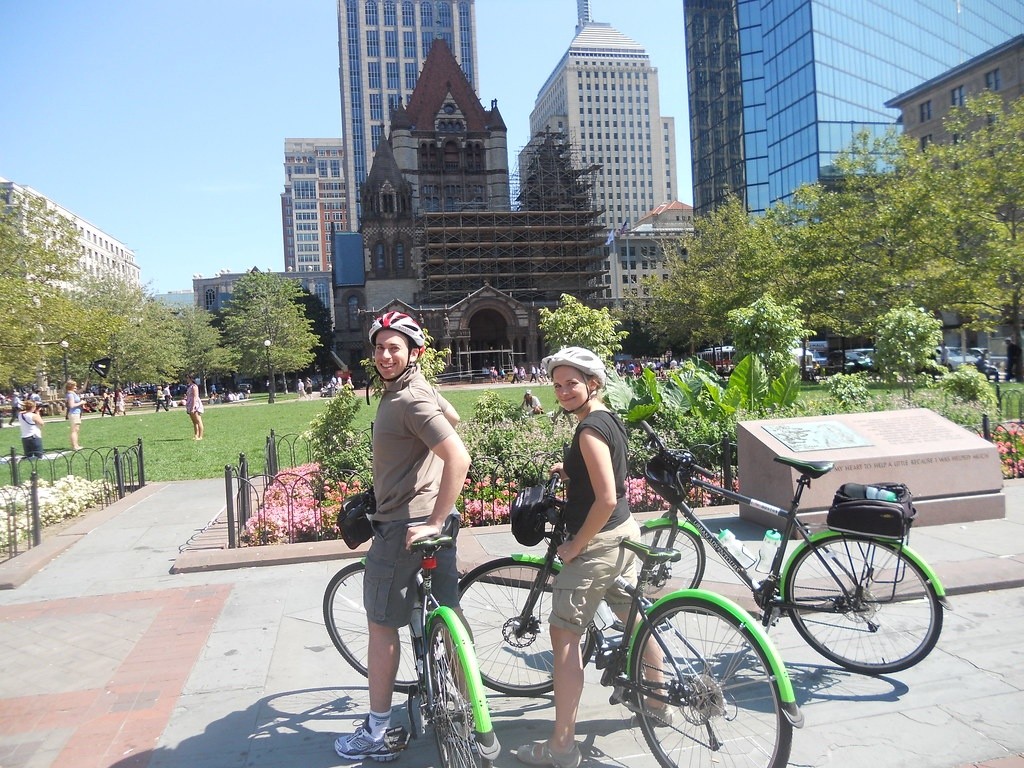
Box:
[722,363,731,379]
[335,311,476,760]
[517,346,674,766]
[1004,337,1022,382]
[811,362,820,381]
[186,375,204,440]
[65,380,86,451]
[306,377,312,397]
[321,375,354,396]
[489,365,549,385]
[941,343,948,366]
[297,379,306,399]
[155,385,187,412]
[980,354,1000,383]
[0,384,43,460]
[520,390,545,414]
[209,383,251,404]
[82,384,156,418]
[616,358,678,378]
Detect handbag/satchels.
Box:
[827,481,918,533]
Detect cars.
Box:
[696,339,1001,382]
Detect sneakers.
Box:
[464,717,478,752]
[334,713,400,762]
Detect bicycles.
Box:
[617,418,955,677]
[455,463,804,768]
[322,488,503,768]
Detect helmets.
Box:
[542,347,606,388]
[369,311,424,348]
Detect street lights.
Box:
[60,341,69,393]
[836,289,846,376]
[264,339,274,397]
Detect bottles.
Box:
[866,486,898,502]
[717,528,759,575]
[408,601,424,683]
[754,527,782,581]
[593,596,615,631]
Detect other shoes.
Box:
[643,697,673,726]
[74,447,82,451]
[516,739,582,768]
